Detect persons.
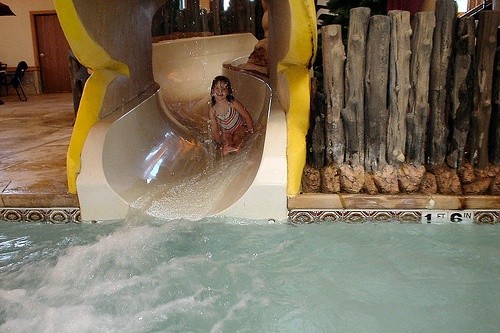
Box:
[208,75,255,156]
[67,46,94,128]
[241,11,271,78]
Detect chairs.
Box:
[0,59,30,101]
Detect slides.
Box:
[76,33,287,229]
